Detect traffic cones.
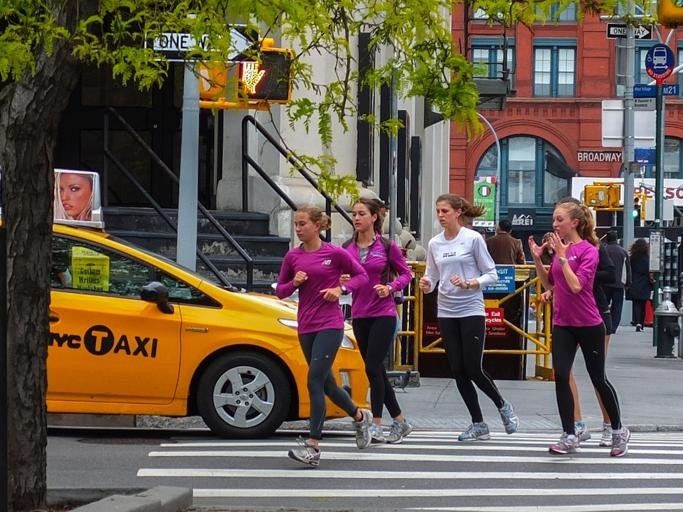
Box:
[644,299,655,324]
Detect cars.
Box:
[45,222,370,440]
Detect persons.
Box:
[419,195,518,441]
[542,232,558,264]
[59,173,90,217]
[626,238,652,332]
[484,219,525,264]
[370,199,407,258]
[528,203,631,456]
[341,197,412,443]
[555,196,612,446]
[276,206,373,467]
[604,230,632,334]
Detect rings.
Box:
[379,291,381,294]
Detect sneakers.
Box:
[549,423,592,455]
[600,423,612,447]
[459,422,490,440]
[611,426,631,456]
[498,402,519,434]
[387,418,412,443]
[352,408,384,449]
[288,435,321,467]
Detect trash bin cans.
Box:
[643,291,654,326]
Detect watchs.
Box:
[387,285,394,294]
[340,284,348,295]
[465,279,471,289]
[559,257,567,265]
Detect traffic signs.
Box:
[144,23,261,63]
[605,23,653,41]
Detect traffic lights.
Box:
[238,50,289,100]
[633,193,642,226]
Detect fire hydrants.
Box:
[653,297,681,358]
